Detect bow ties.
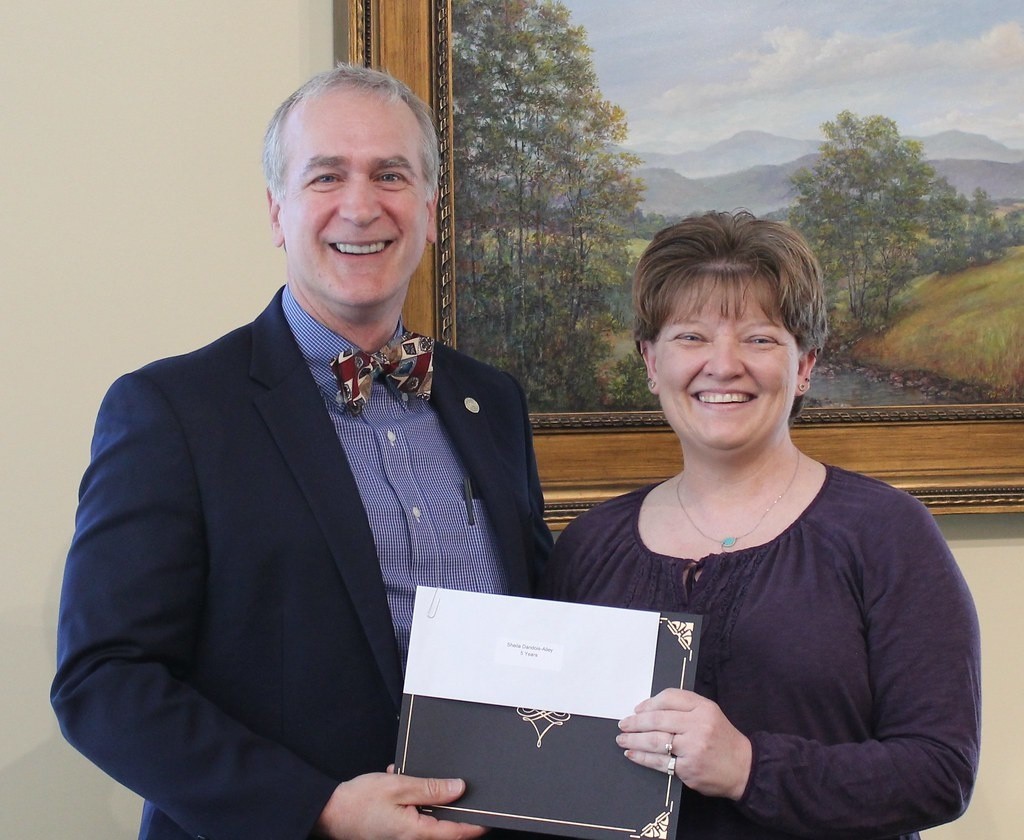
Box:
[330,330,435,418]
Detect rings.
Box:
[668,756,676,775]
[665,734,675,756]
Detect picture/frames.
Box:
[328,0,1024,527]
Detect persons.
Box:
[50,68,553,840]
[547,211,981,839]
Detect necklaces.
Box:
[677,450,800,546]
